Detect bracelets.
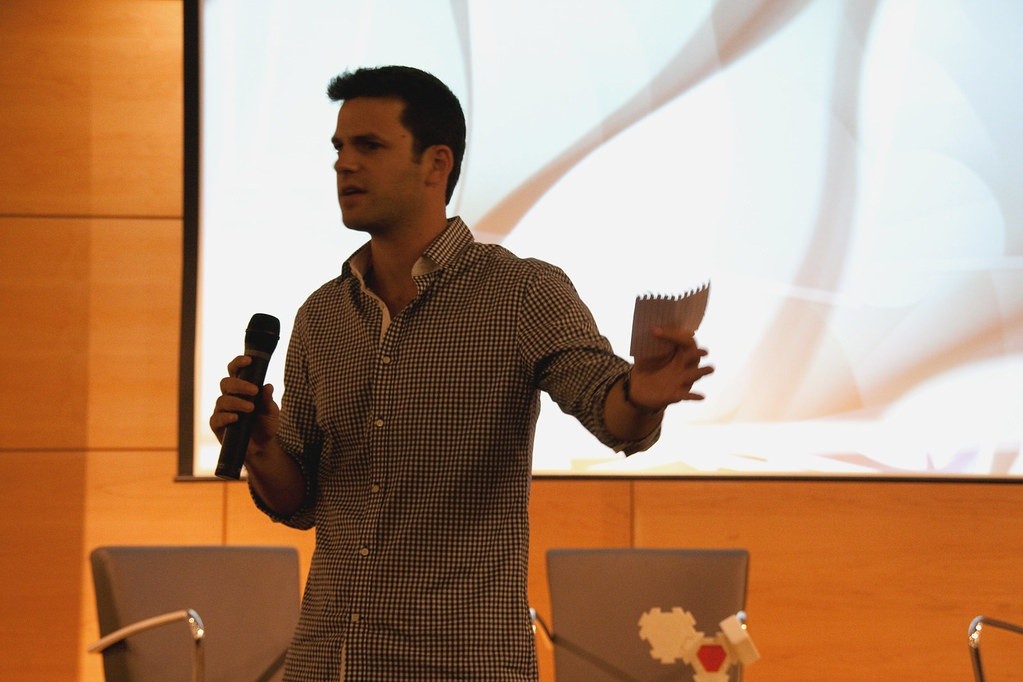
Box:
[622,366,666,417]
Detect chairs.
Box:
[528,547,751,682]
[86,544,302,681]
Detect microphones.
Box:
[212,314,280,481]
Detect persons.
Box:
[210,65,714,682]
